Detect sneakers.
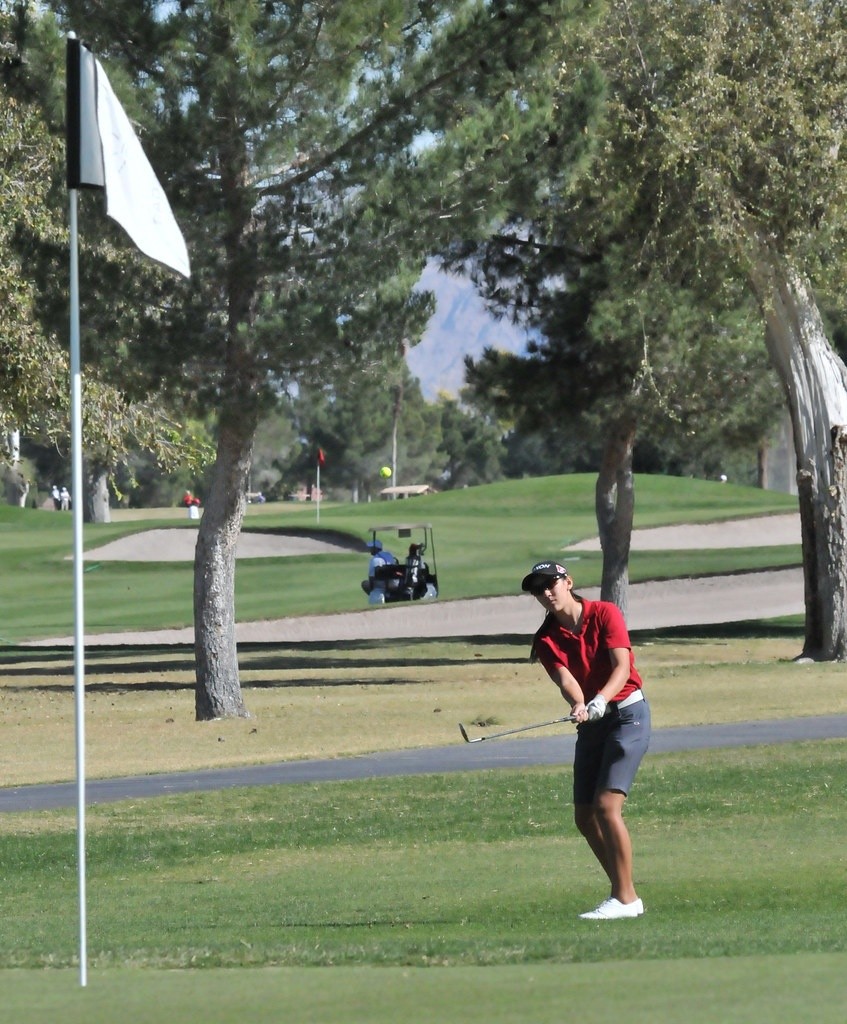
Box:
[578,896,636,919]
[636,898,644,914]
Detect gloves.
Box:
[586,694,608,721]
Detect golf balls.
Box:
[379,466,392,479]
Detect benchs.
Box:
[364,562,429,602]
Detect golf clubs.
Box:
[458,715,578,743]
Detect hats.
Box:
[365,539,382,549]
[521,559,566,593]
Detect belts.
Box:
[603,689,644,713]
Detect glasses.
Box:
[531,573,565,597]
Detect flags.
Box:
[64,40,192,280]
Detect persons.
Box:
[189,498,201,519]
[53,485,71,511]
[520,560,652,919]
[257,492,266,503]
[184,491,194,507]
[361,540,399,596]
